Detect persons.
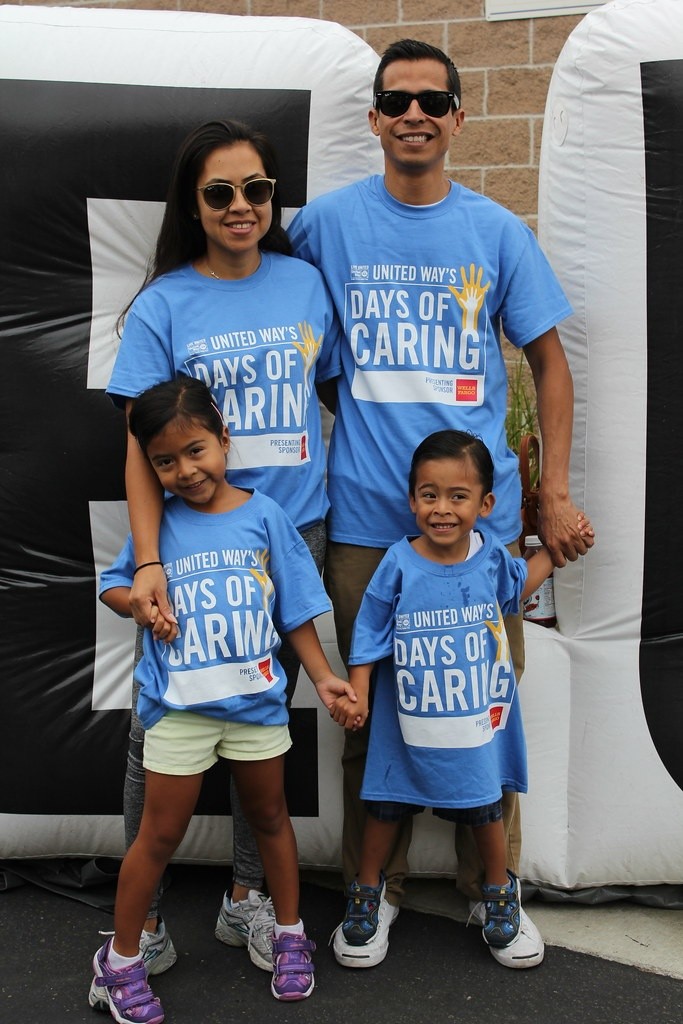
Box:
[329,430,594,934]
[285,39,592,968]
[93,376,361,1024]
[91,123,342,1004]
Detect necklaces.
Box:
[202,257,220,279]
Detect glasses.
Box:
[373,89,460,118]
[196,178,276,211]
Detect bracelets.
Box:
[133,562,163,575]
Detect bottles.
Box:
[521,535,558,628]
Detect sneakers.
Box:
[328,898,400,968]
[271,927,316,1001]
[215,889,276,972]
[88,915,177,1012]
[341,873,386,946]
[92,938,164,1024]
[481,873,522,947]
[467,879,545,967]
